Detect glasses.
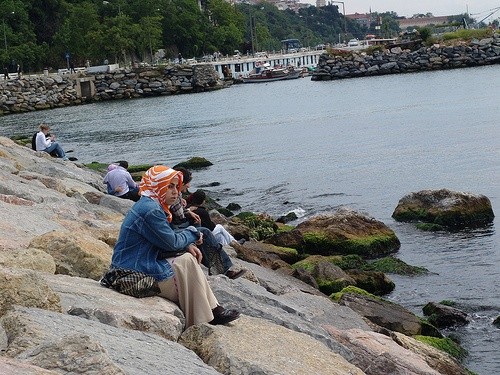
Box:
[168,183,180,189]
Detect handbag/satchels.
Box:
[100,269,162,299]
[204,243,225,275]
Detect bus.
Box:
[280,39,302,54]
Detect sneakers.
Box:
[225,269,246,280]
[208,303,240,325]
[238,238,245,245]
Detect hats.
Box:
[107,165,117,171]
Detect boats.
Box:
[242,62,304,83]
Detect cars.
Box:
[349,39,358,47]
[315,44,326,49]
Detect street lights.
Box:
[3,11,14,52]
[248,6,265,56]
[331,1,348,47]
[147,8,160,63]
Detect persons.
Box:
[70,60,76,74]
[104,58,109,65]
[18,64,21,79]
[109,165,241,330]
[213,51,222,62]
[103,161,141,202]
[32,124,70,161]
[4,66,10,80]
[178,52,184,64]
[167,167,246,279]
[154,50,166,64]
